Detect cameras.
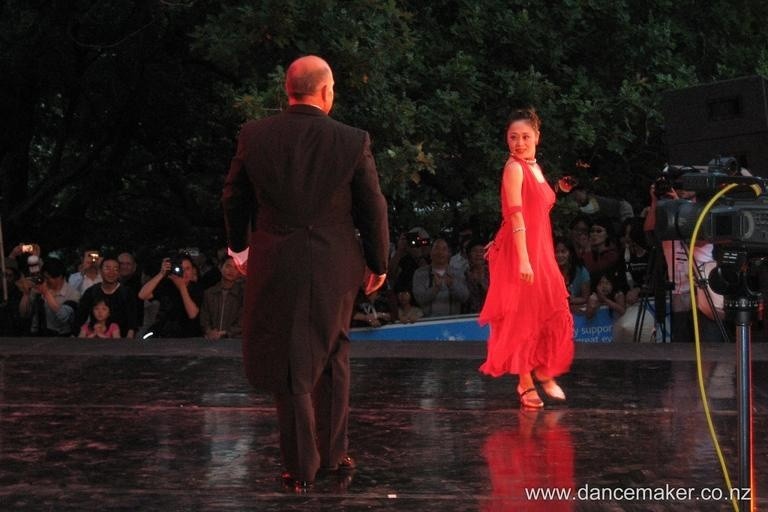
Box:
[408,232,432,248]
[32,275,44,285]
[88,250,105,263]
[653,179,671,198]
[22,244,33,252]
[167,264,184,277]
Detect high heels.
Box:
[543,380,567,399]
[515,387,544,407]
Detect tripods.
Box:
[632,240,730,345]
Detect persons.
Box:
[224,56,389,488]
[0,169,764,339]
[477,105,575,406]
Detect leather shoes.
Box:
[283,474,314,490]
[327,455,355,471]
